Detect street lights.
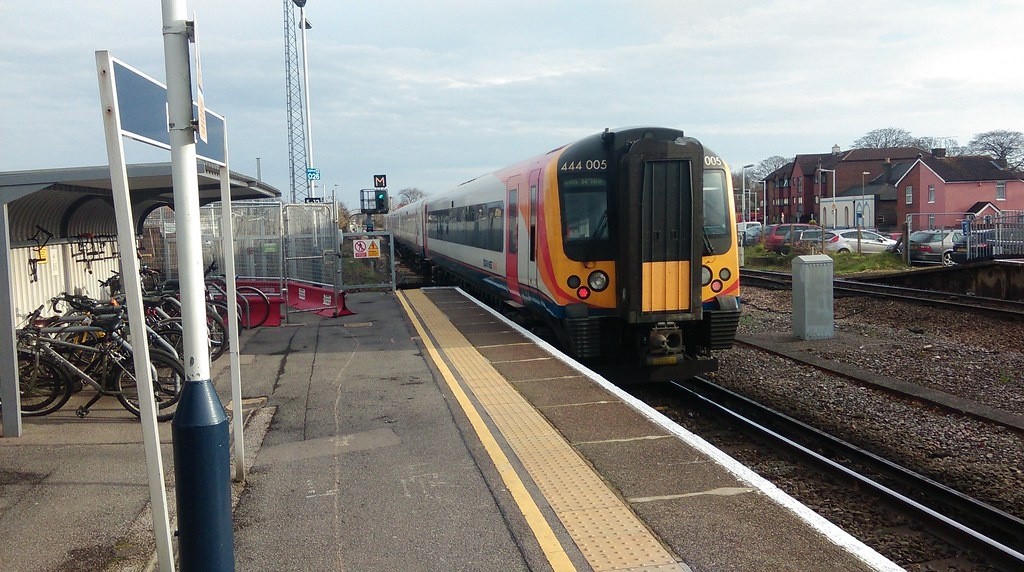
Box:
[817,168,837,229]
[741,162,755,240]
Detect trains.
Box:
[379,125,746,390]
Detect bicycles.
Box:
[16,254,274,427]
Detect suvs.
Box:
[735,220,829,259]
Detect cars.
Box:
[817,228,905,260]
[910,228,984,267]
[951,226,1024,264]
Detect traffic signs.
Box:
[375,189,389,213]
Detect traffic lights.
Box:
[373,174,387,187]
